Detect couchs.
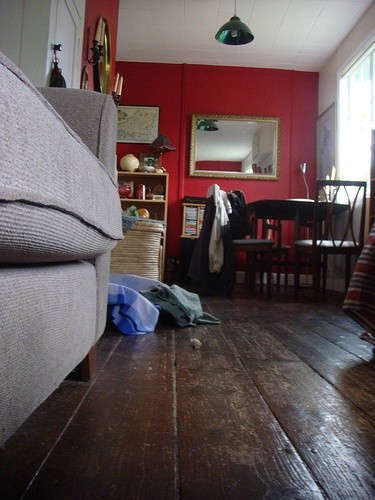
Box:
[0,49,125,448]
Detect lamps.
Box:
[214,0,254,46]
[197,119,218,131]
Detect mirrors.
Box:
[195,119,275,176]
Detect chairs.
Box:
[292,180,367,302]
[259,219,290,290]
[208,184,275,294]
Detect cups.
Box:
[132,183,151,201]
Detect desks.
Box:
[247,200,350,302]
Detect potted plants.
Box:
[165,255,177,272]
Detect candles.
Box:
[95,16,106,46]
[113,72,119,92]
[118,76,123,95]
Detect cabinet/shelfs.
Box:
[117,171,170,223]
[180,196,207,272]
